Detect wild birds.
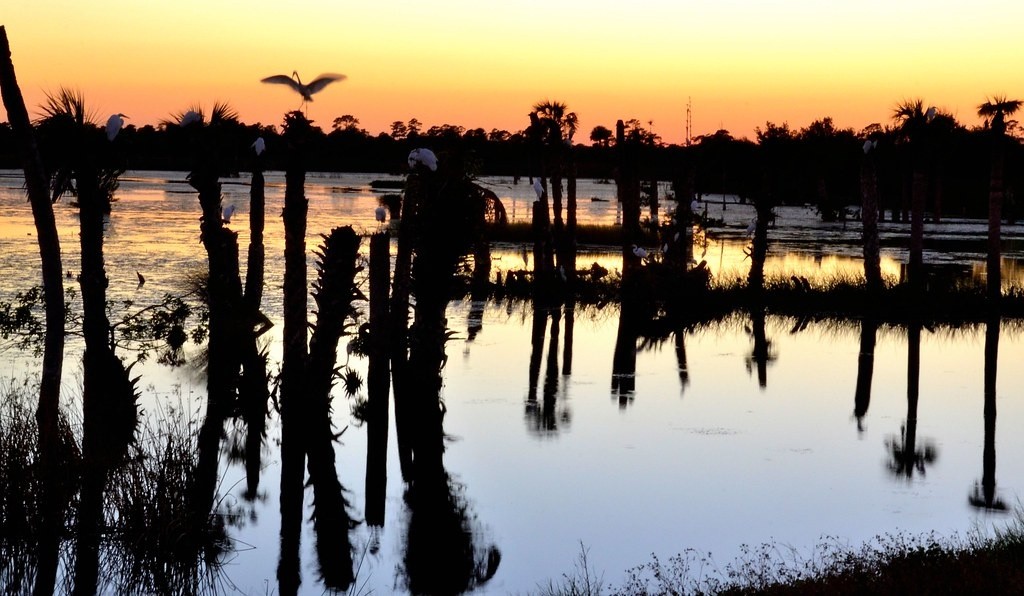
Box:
[180,111,202,127]
[105,113,129,142]
[375,207,386,232]
[260,70,347,120]
[862,140,872,155]
[407,147,438,172]
[251,136,265,156]
[927,106,936,121]
[666,188,757,238]
[223,205,236,224]
[632,244,648,264]
[534,177,544,202]
